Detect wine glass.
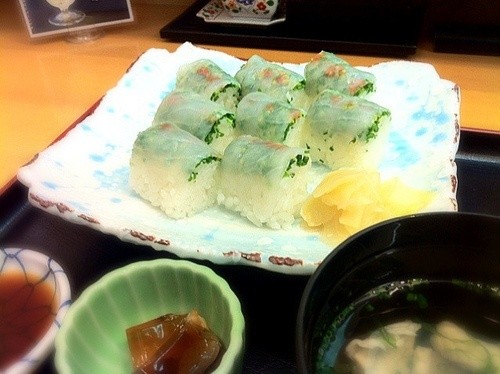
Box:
[46,0,86,27]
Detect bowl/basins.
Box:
[295,213,500,374]
[220,0,279,21]
[52,258,246,374]
[0,247,72,374]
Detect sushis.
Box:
[128,52,392,229]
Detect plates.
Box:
[196,1,286,26]
[16,41,462,282]
